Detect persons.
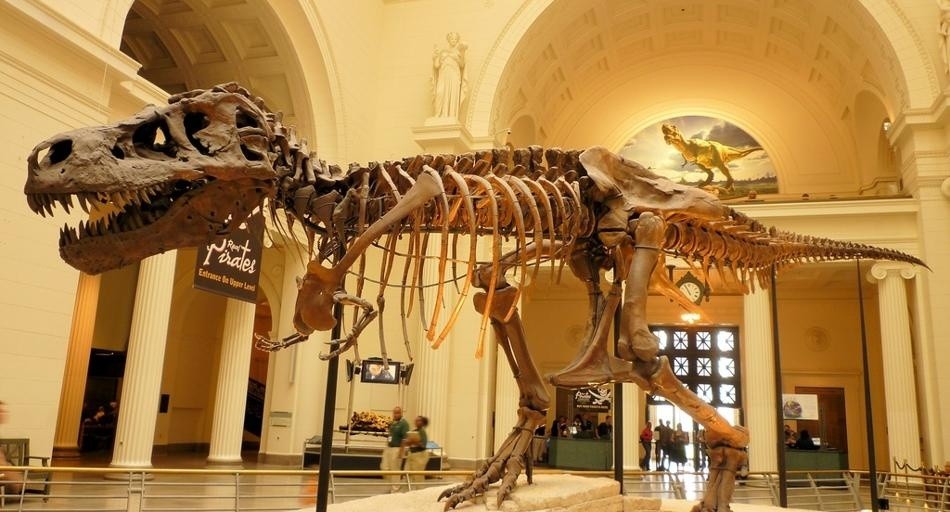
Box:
[378,405,409,493]
[536,413,813,472]
[0,400,28,505]
[401,415,429,490]
[79,399,119,454]
[365,364,394,382]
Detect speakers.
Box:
[160,394,169,413]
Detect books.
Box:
[405,430,421,447]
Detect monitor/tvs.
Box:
[811,437,820,445]
[360,359,400,385]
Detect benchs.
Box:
[0,438,55,504]
